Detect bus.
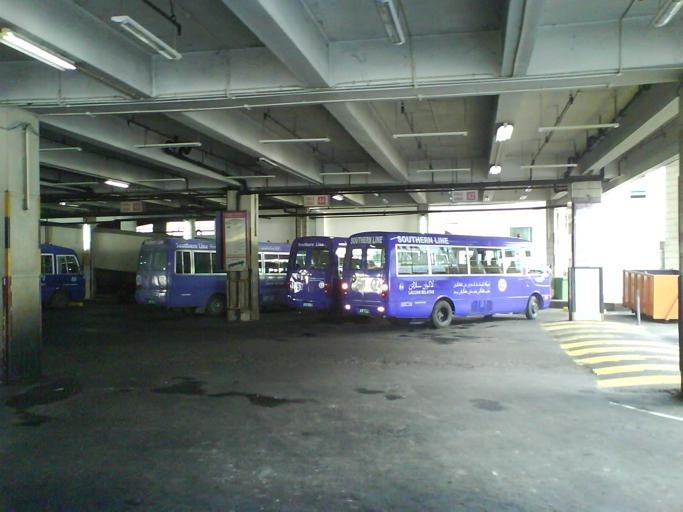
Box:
[133,235,297,319]
[38,241,86,311]
[337,229,555,331]
[280,235,349,323]
